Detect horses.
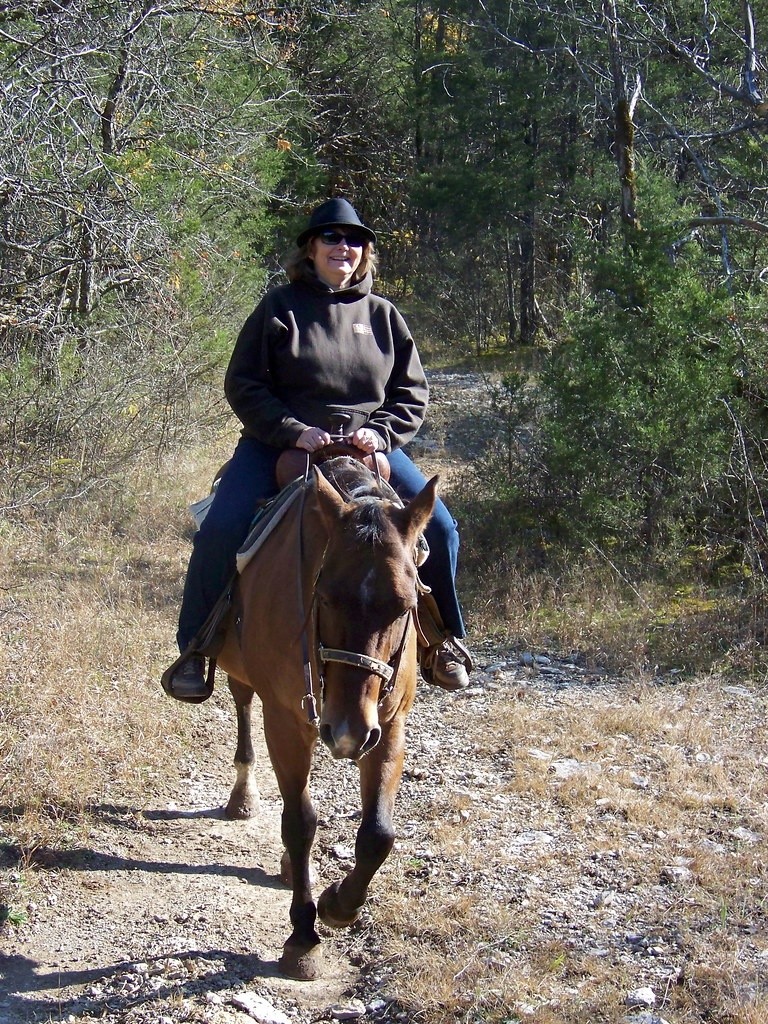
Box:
[213,454,441,982]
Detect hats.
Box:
[297,198,378,249]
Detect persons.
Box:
[161,197,476,704]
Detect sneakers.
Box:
[431,642,470,690]
[173,651,207,697]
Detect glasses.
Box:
[313,231,365,247]
